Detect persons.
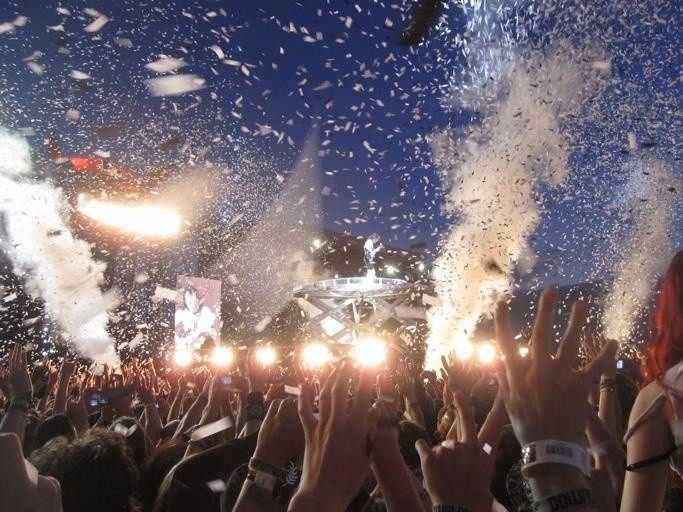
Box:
[1,251,683,512]
[364,232,380,280]
